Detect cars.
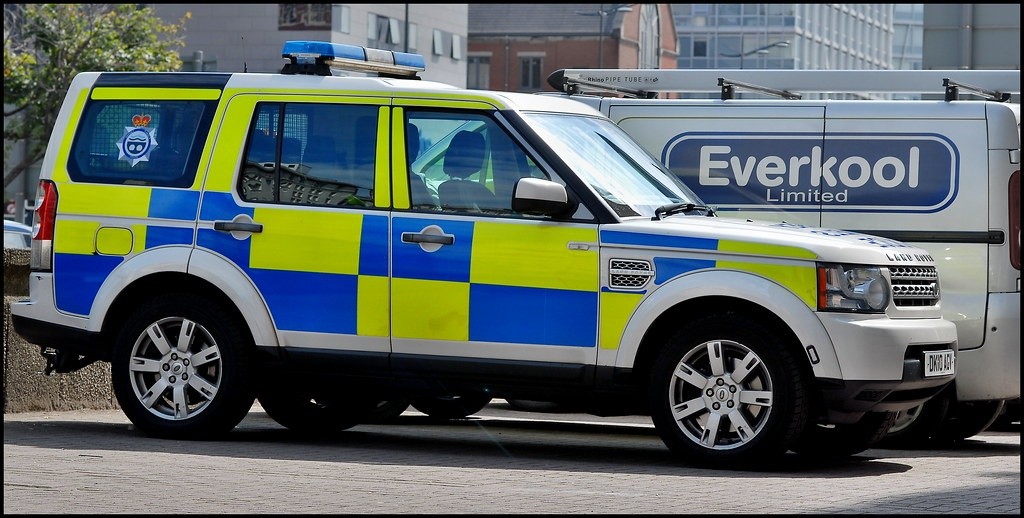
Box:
[4,218,32,251]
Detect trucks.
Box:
[404,69,1022,446]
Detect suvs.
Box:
[7,40,959,474]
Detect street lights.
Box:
[575,3,633,70]
[719,32,790,70]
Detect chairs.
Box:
[248,129,301,164]
[405,122,436,208]
[302,132,352,184]
[149,124,186,164]
[438,130,499,208]
